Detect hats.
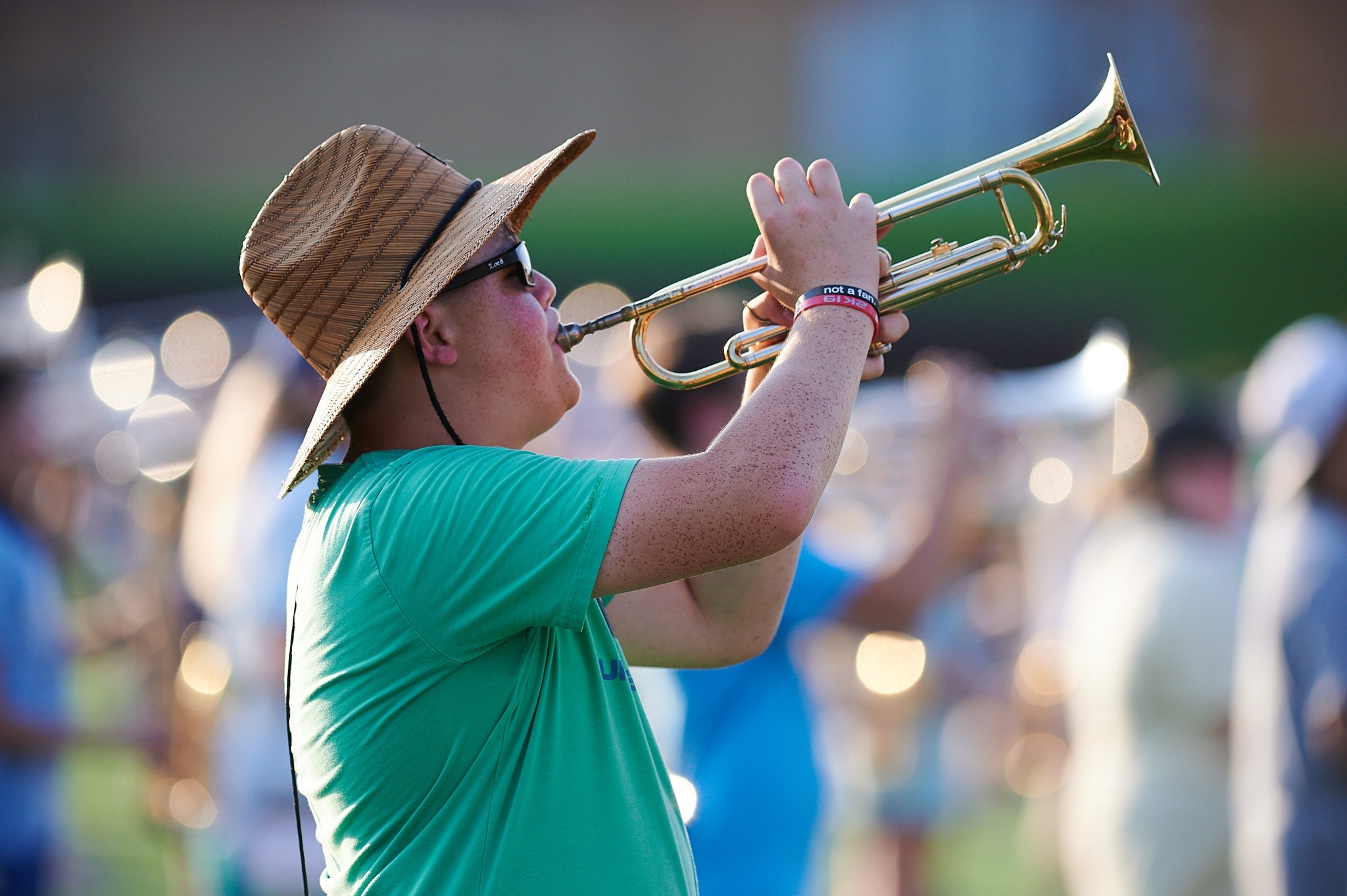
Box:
[238,124,598,499]
[1237,312,1347,504]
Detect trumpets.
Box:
[551,51,1163,391]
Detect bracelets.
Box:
[795,283,881,323]
[792,294,879,343]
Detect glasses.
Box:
[430,238,538,290]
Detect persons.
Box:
[1,287,1347,896]
[242,122,908,895]
